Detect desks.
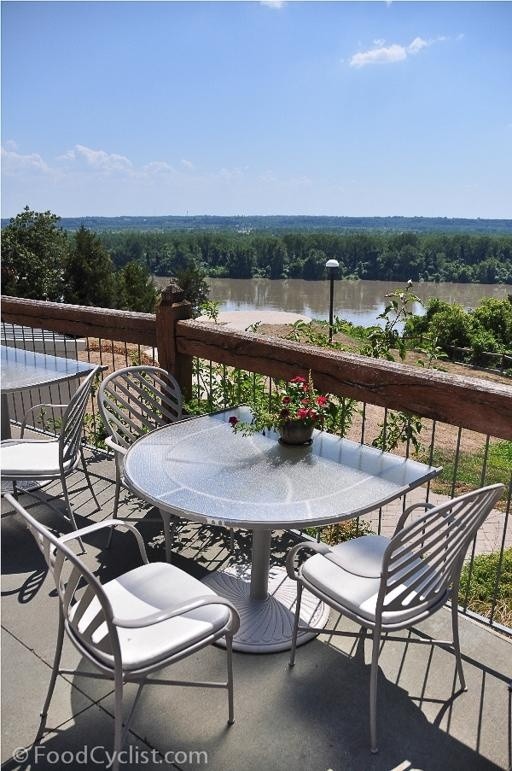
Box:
[122,401,443,657]
[0,340,110,496]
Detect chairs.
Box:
[1,489,243,771]
[95,361,241,565]
[282,481,509,755]
[0,362,103,554]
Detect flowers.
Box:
[224,364,331,439]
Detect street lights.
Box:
[324,258,340,345]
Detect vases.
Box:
[277,411,318,446]
